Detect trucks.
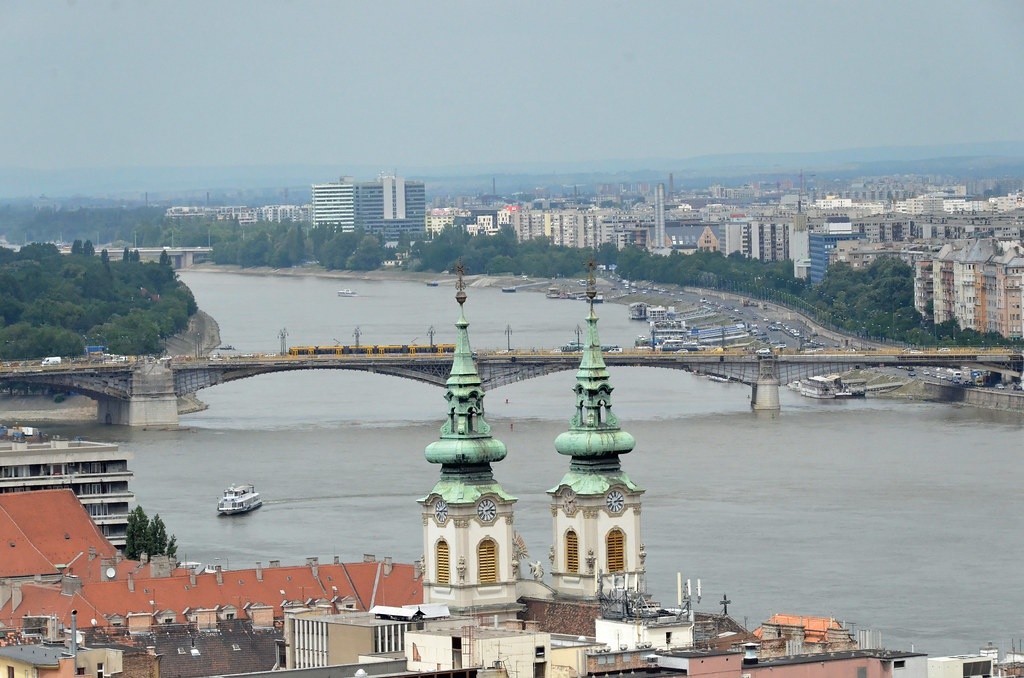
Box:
[42,355,62,364]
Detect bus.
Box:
[663,339,684,351]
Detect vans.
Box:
[608,347,623,353]
[685,343,698,351]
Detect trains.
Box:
[289,343,456,355]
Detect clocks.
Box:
[606,490,624,512]
[565,490,577,514]
[478,499,497,522]
[435,500,448,522]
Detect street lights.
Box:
[504,322,511,354]
[650,323,656,352]
[720,326,727,354]
[277,326,287,358]
[427,323,435,350]
[351,326,361,353]
[575,325,583,353]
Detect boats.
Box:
[787,375,835,398]
[215,481,264,518]
[338,288,360,296]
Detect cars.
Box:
[910,348,924,354]
[700,290,854,350]
[937,348,952,353]
[898,363,1023,391]
[756,348,772,353]
